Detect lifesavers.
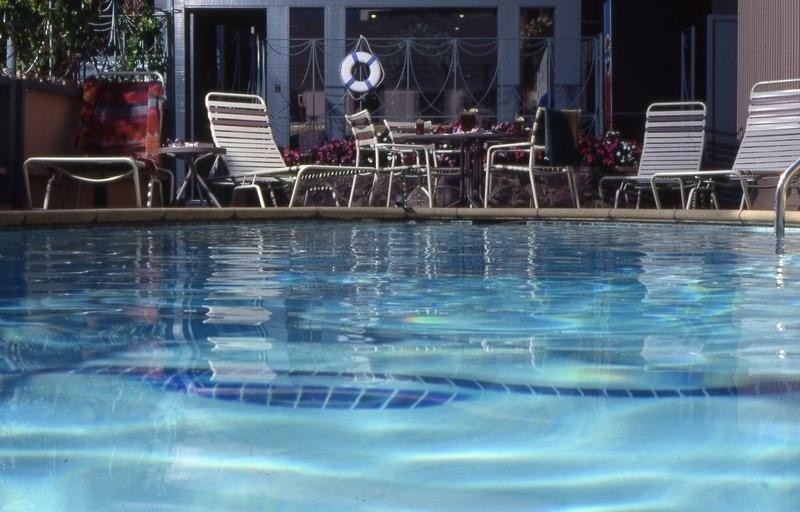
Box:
[340,51,382,93]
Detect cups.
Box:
[414,119,425,136]
[513,120,523,132]
